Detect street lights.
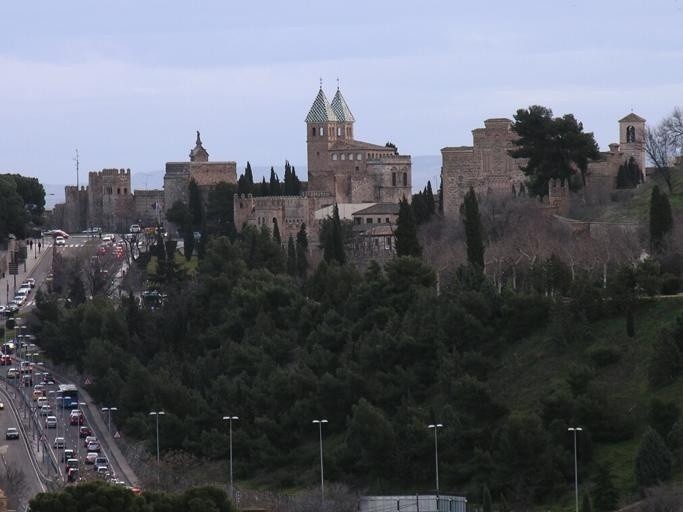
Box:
[568,427,583,512]
[312,420,329,504]
[223,416,239,488]
[428,424,444,494]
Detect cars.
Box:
[0,278,167,495]
[41,224,168,277]
